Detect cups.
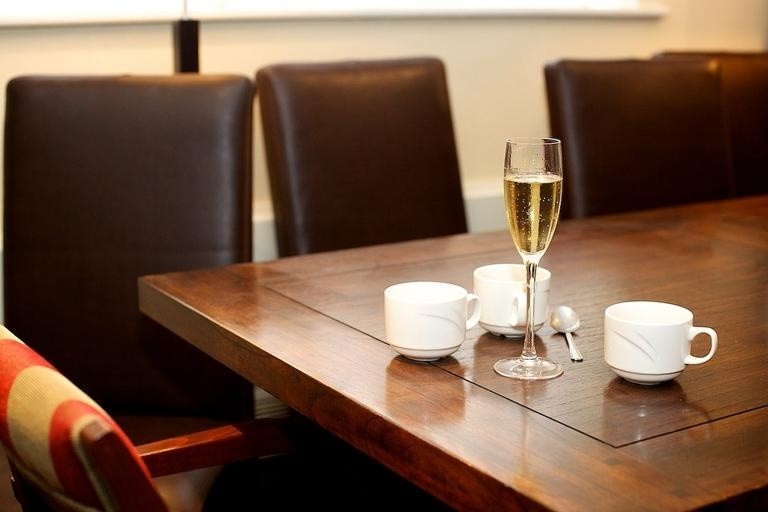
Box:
[473,263,552,338]
[603,301,718,385]
[384,281,482,361]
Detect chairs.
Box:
[543,51,765,222]
[1,325,252,511]
[2,56,470,411]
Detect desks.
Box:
[139,197,767,511]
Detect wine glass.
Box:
[493,136,564,380]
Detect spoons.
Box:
[550,304,584,361]
